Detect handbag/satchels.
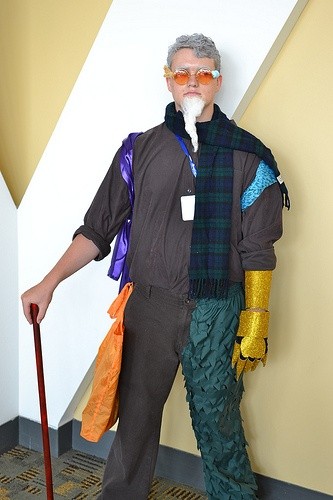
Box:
[108,219,132,281]
[79,282,134,442]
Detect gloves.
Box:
[232,271,272,381]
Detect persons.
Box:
[21,34,292,500]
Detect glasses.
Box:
[163,65,220,85]
